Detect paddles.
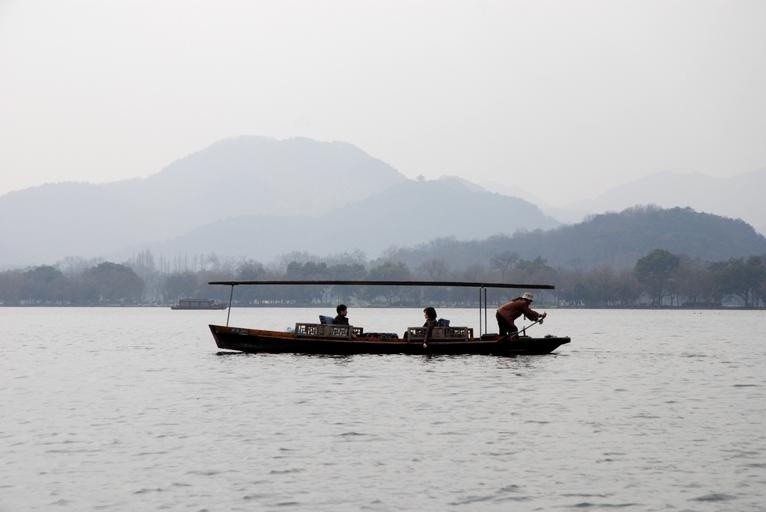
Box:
[495,312,546,349]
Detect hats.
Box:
[522,292,533,300]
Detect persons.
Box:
[332,304,350,325]
[420,306,439,336]
[496,292,548,337]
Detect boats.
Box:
[206,277,572,354]
[171,299,228,309]
